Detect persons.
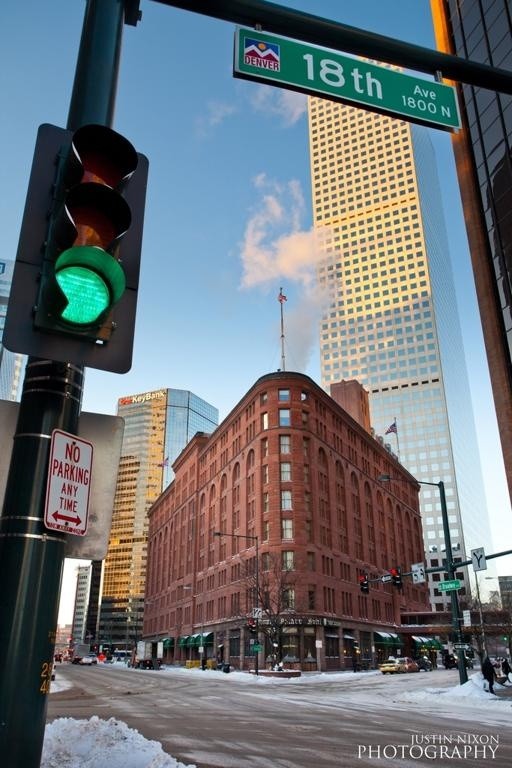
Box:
[482,657,512,694]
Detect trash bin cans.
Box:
[51,664,56,681]
[223,663,230,673]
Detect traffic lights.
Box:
[2,124,149,374]
[389,567,402,586]
[359,573,369,594]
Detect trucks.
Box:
[127,641,166,669]
[71,644,91,665]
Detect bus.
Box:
[113,651,132,661]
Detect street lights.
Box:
[183,586,203,668]
[379,476,469,686]
[213,532,258,676]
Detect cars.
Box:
[377,655,433,674]
[79,653,97,664]
[444,653,473,668]
